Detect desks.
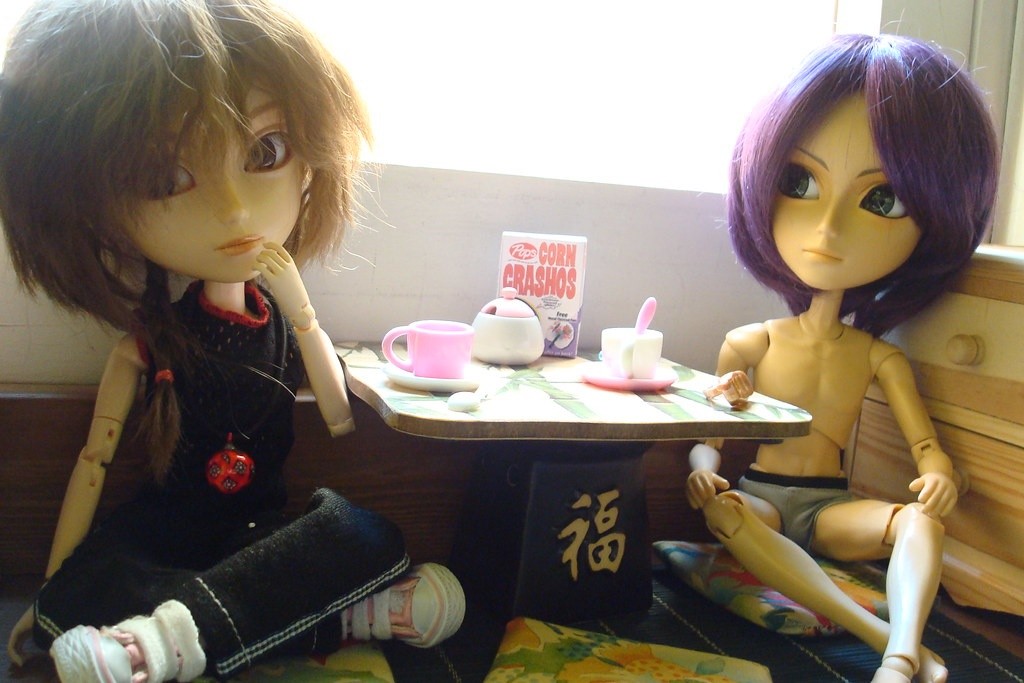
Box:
[336,338,815,625]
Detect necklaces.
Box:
[182,283,289,496]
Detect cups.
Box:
[381,319,477,380]
[470,286,546,366]
[600,327,664,382]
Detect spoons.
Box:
[446,365,499,413]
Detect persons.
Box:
[0,0,466,683]
[683,32,1002,683]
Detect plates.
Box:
[383,360,488,394]
[580,358,678,393]
